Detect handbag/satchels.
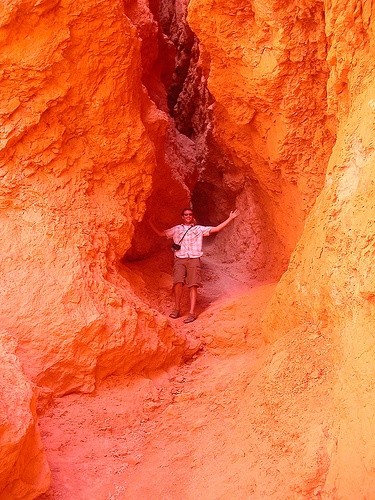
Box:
[173,244,181,250]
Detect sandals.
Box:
[169,309,179,318]
[184,313,198,323]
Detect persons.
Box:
[146,207,240,324]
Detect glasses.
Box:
[184,213,192,216]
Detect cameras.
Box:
[172,243,181,250]
